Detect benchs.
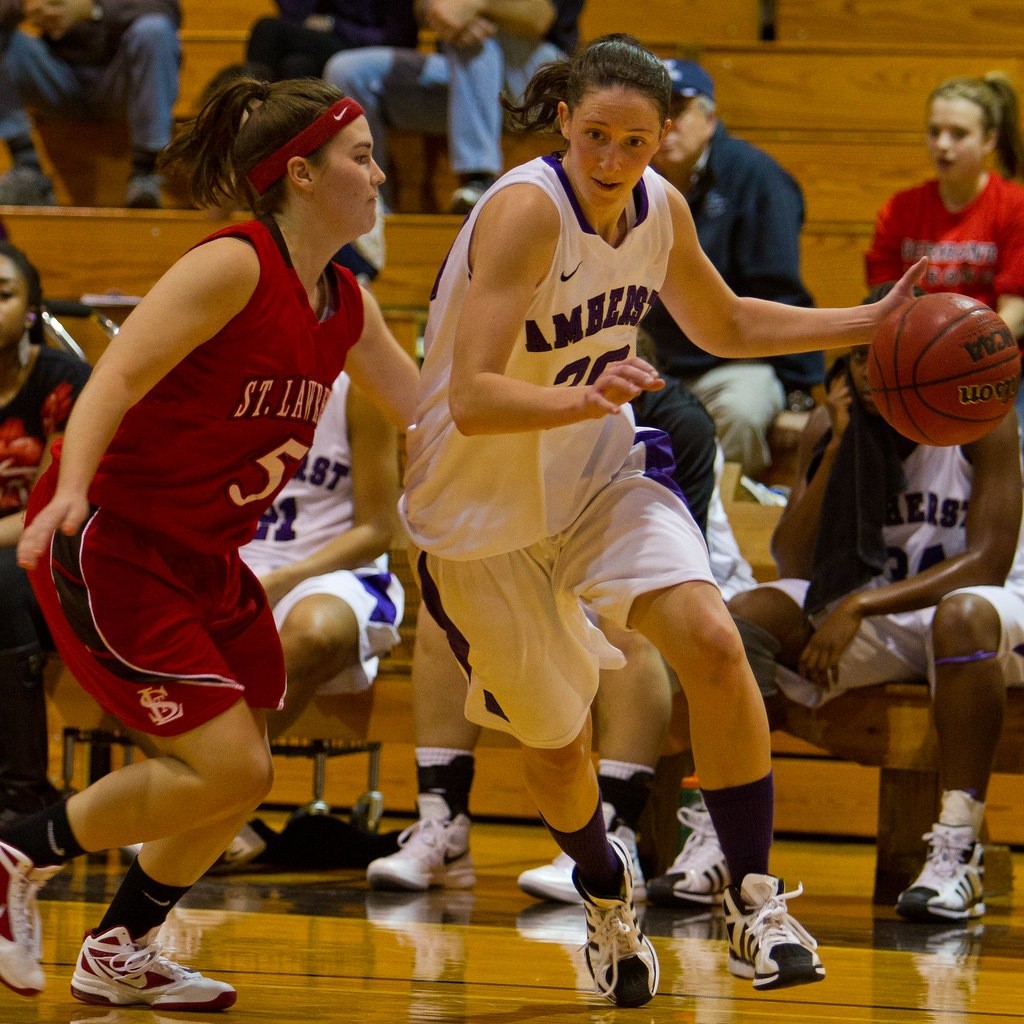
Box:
[1,1,1024,915]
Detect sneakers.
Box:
[71,926,238,1011]
[571,832,659,1006]
[1,840,72,997]
[722,872,828,992]
[895,823,989,920]
[519,799,648,904]
[641,906,728,955]
[68,1007,197,1024]
[366,889,475,981]
[152,882,263,958]
[367,814,479,896]
[645,785,731,906]
[893,923,986,999]
[516,902,596,994]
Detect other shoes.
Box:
[329,199,385,283]
[1,164,59,206]
[448,175,496,217]
[117,809,269,873]
[124,174,162,211]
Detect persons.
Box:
[402,32,927,1010]
[2,76,421,1007]
[1,0,1024,922]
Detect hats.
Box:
[658,57,716,101]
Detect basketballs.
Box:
[867,292,1021,447]
